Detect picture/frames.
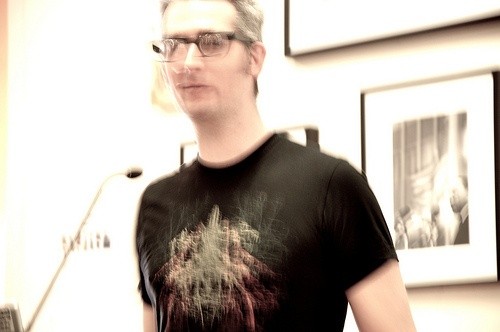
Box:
[358,66,500,288]
[282,0,500,62]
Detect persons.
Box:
[129,1,415,332]
